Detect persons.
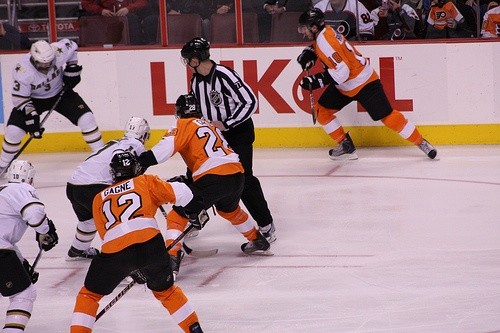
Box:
[0,159,59,333]
[139,94,270,282]
[181,37,278,244]
[166,0,500,45]
[69,151,210,333]
[76,0,148,46]
[0,38,104,170]
[298,7,437,159]
[66,116,151,259]
[0,20,32,49]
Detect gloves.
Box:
[35,219,59,252]
[62,65,82,89]
[300,72,328,90]
[25,109,45,138]
[297,47,318,72]
[136,150,157,176]
[166,175,189,184]
[182,195,210,229]
[129,270,147,285]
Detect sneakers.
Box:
[417,138,439,161]
[240,231,270,255]
[184,223,199,237]
[328,131,358,160]
[258,223,279,248]
[169,250,185,281]
[65,246,99,261]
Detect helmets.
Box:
[7,159,34,185]
[298,8,326,31]
[175,95,203,118]
[30,40,55,63]
[181,37,211,62]
[125,116,150,145]
[110,152,137,177]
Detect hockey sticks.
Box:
[32,248,46,270]
[307,66,319,121]
[0,89,66,181]
[157,205,220,257]
[94,220,195,318]
